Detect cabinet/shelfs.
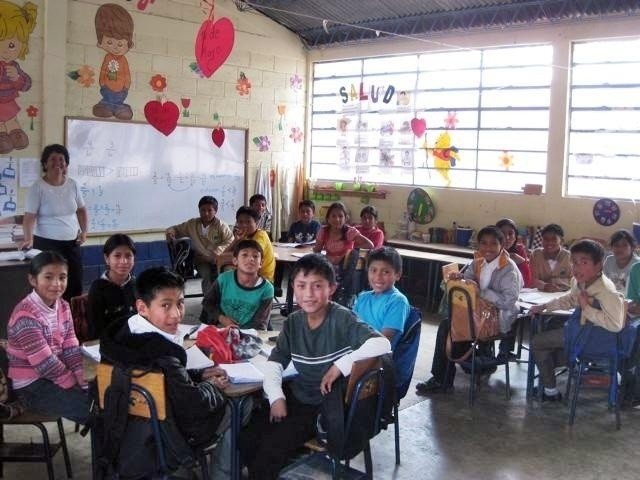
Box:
[310,186,387,200]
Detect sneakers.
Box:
[417,379,453,394]
[534,388,561,401]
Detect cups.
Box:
[456,228,477,247]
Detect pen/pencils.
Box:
[185,326,198,337]
[459,262,468,274]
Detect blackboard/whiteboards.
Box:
[64,116,249,237]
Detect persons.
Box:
[527,239,625,402]
[529,223,573,292]
[351,245,408,351]
[101,268,234,480]
[165,196,235,324]
[91,4,135,121]
[625,262,640,315]
[352,206,384,250]
[239,253,392,480]
[601,229,640,289]
[201,239,275,331]
[234,206,275,287]
[250,194,272,237]
[7,252,94,428]
[88,232,136,339]
[0,0,37,153]
[287,201,321,242]
[495,219,531,288]
[18,143,88,301]
[313,203,374,266]
[415,224,525,395]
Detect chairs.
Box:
[333,247,377,307]
[165,235,206,302]
[91,362,219,478]
[71,294,101,359]
[562,302,627,431]
[441,278,514,404]
[390,306,423,464]
[1,344,76,480]
[280,352,394,480]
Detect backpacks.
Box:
[173,239,195,277]
[94,366,196,480]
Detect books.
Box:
[79,344,100,363]
[178,324,197,338]
[292,252,313,258]
[219,357,297,383]
[275,240,317,248]
[519,292,557,305]
[186,345,214,370]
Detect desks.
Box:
[79,324,298,479]
[1,260,39,340]
[223,241,330,318]
[509,291,581,402]
[386,235,481,313]
